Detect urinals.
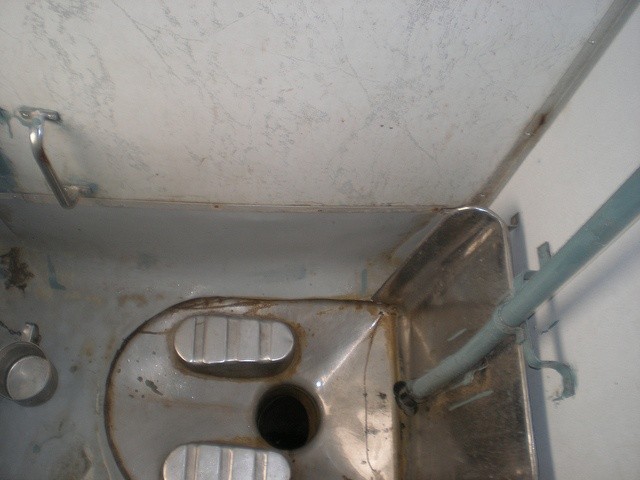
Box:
[0,197,539,480]
